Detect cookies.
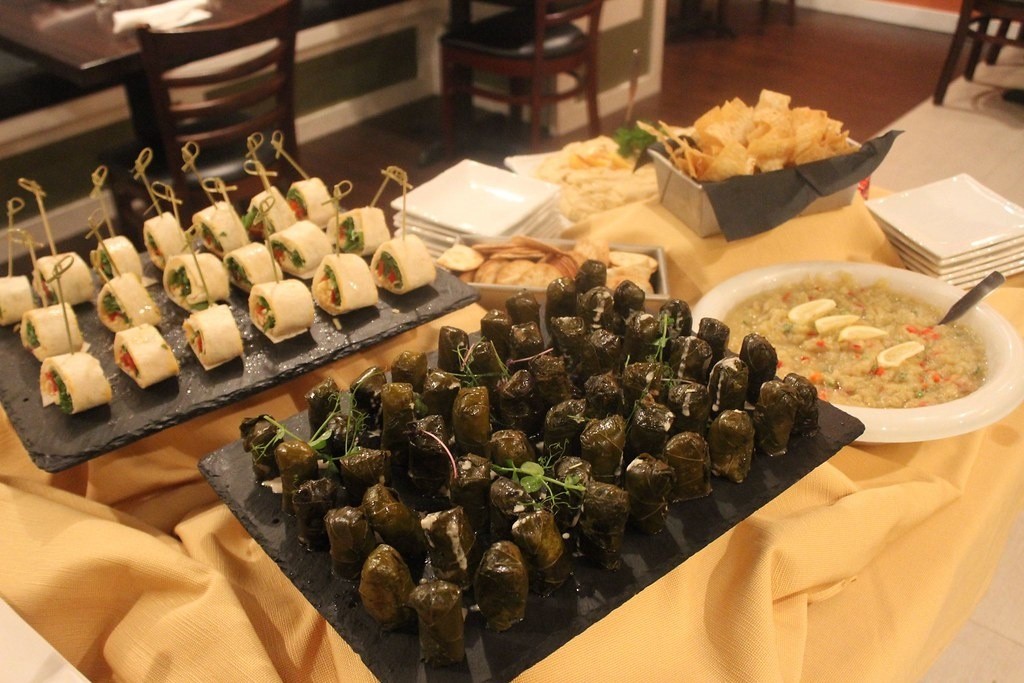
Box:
[436,234,662,297]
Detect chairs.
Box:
[930,0,1024,106]
[97,0,306,246]
[437,0,603,153]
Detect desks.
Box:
[0,46,1023,682]
[0,0,423,135]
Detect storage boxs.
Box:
[638,130,905,241]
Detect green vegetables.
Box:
[612,118,670,160]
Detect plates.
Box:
[864,172,1024,291]
[390,150,572,275]
[177,8,211,28]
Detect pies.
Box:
[0,176,437,415]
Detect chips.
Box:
[681,88,861,184]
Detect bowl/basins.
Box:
[455,234,670,312]
[689,261,1024,445]
[647,132,862,238]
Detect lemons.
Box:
[787,298,926,368]
